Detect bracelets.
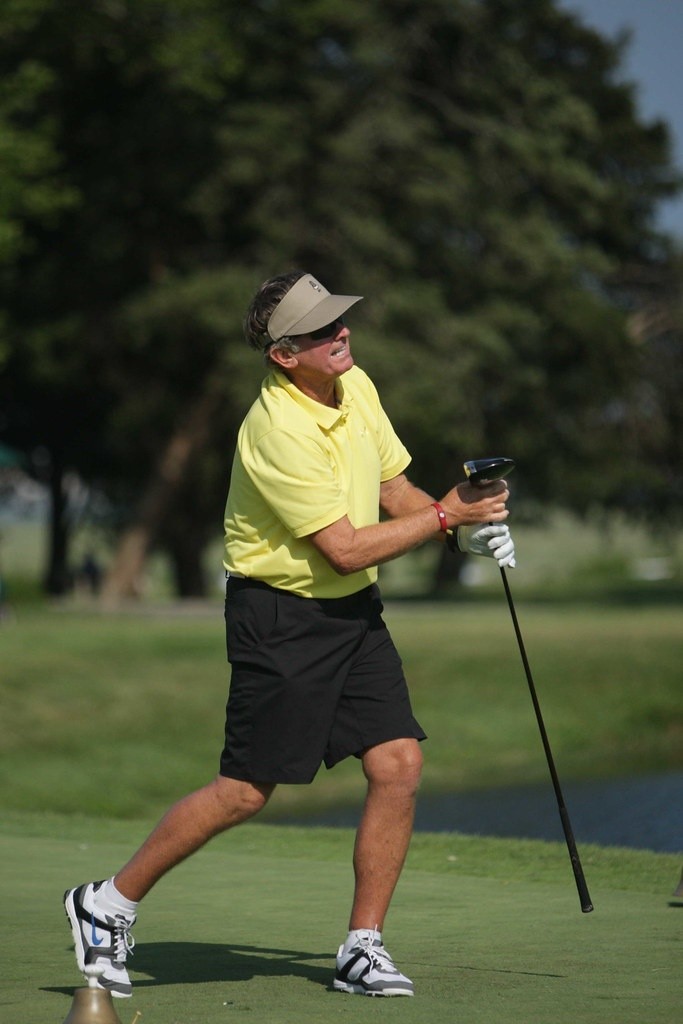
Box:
[430,502,454,535]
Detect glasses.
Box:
[309,314,345,341]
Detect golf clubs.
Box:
[464,456,594,915]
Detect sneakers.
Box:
[63,879,139,999]
[333,924,414,998]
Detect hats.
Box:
[256,273,365,349]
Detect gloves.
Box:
[457,521,517,568]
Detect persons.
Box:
[62,273,519,1000]
